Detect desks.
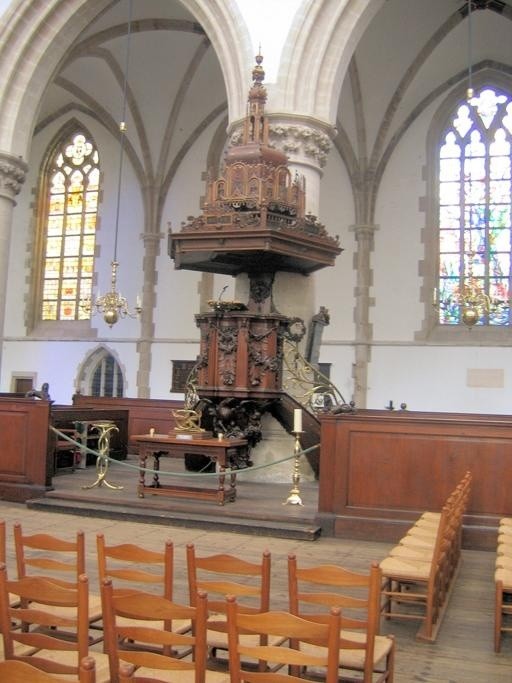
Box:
[130,434,249,505]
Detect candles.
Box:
[218,433,223,438]
[294,408,302,433]
[150,428,155,433]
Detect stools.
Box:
[73,420,112,468]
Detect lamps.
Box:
[74,1,143,331]
[431,0,508,331]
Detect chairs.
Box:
[184,541,288,673]
[97,575,241,683]
[223,593,342,683]
[96,532,194,662]
[50,429,78,475]
[289,553,396,683]
[1,652,96,683]
[374,472,511,655]
[0,563,133,682]
[14,523,104,652]
